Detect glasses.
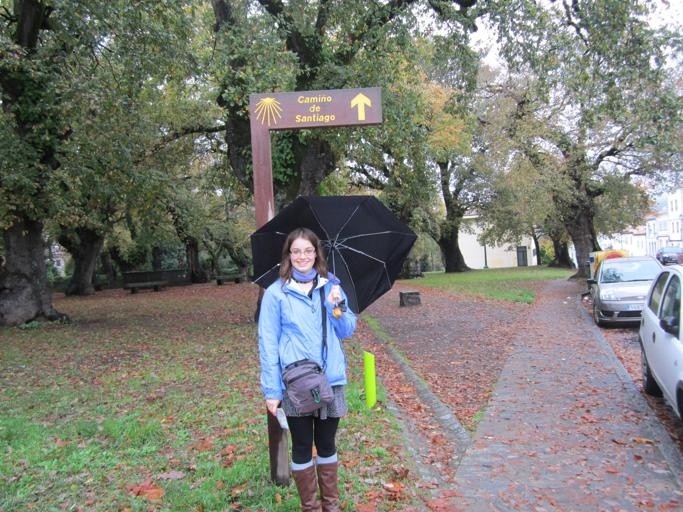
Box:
[288,248,316,256]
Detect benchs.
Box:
[216,274,244,285]
[124,281,168,293]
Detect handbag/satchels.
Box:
[279,356,336,415]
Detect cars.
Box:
[586,241,682,430]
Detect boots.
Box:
[316,450,343,512]
[289,457,323,512]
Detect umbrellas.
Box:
[249,194,419,320]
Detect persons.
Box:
[255,226,359,511]
